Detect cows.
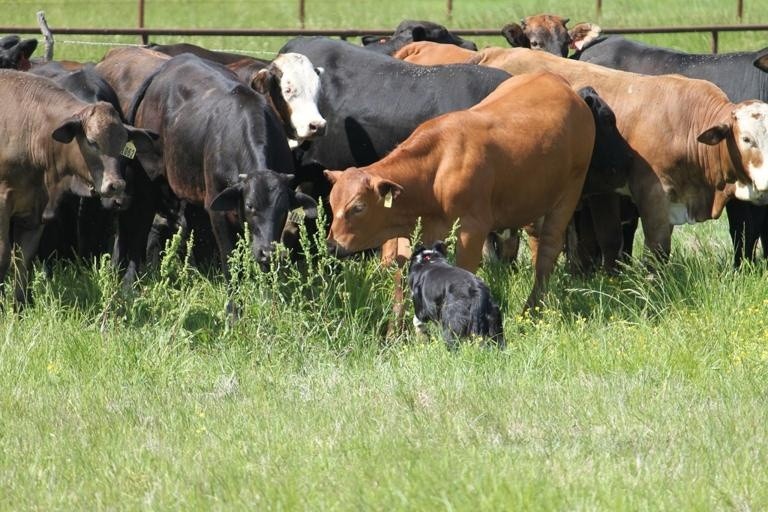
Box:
[1,13,767,332]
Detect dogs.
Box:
[407,240,506,355]
